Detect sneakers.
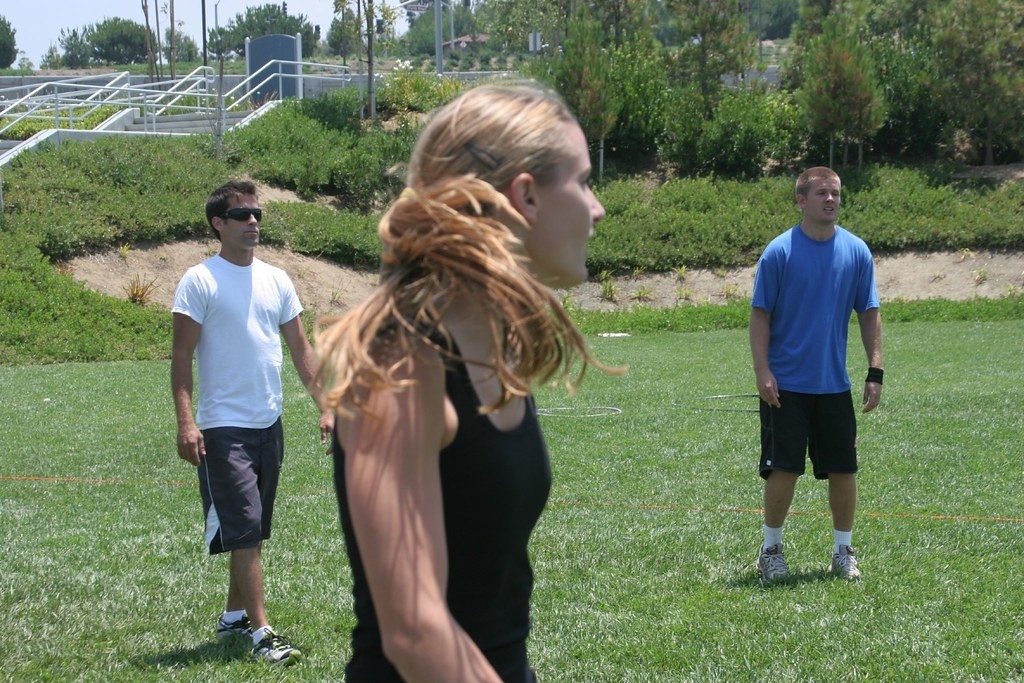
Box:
[217,614,252,638]
[756,543,791,587]
[832,544,863,580]
[252,628,302,667]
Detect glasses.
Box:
[217,207,262,221]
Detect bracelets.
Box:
[865,367,884,385]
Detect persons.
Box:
[308,83,605,683]
[170,180,337,665]
[749,167,882,581]
